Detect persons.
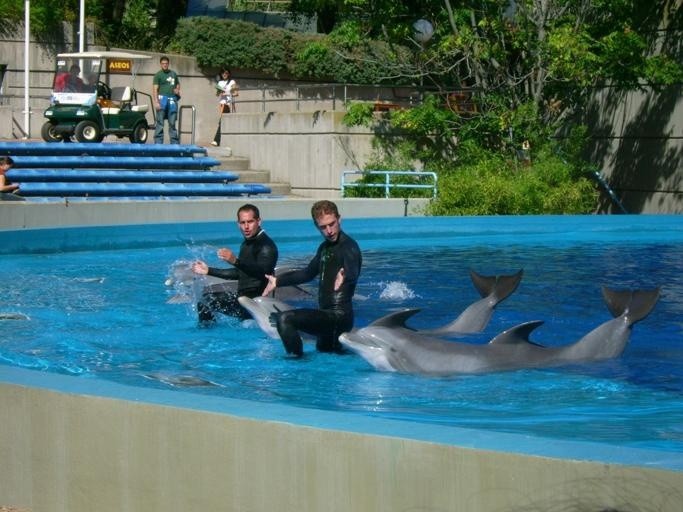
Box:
[209,67,238,146]
[0,157,19,191]
[54,64,82,142]
[152,56,181,144]
[261,200,362,359]
[191,204,278,329]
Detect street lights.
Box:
[410,18,432,104]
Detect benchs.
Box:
[1,142,272,200]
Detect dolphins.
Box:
[238,266,523,340]
[337,282,660,375]
[165,262,340,305]
